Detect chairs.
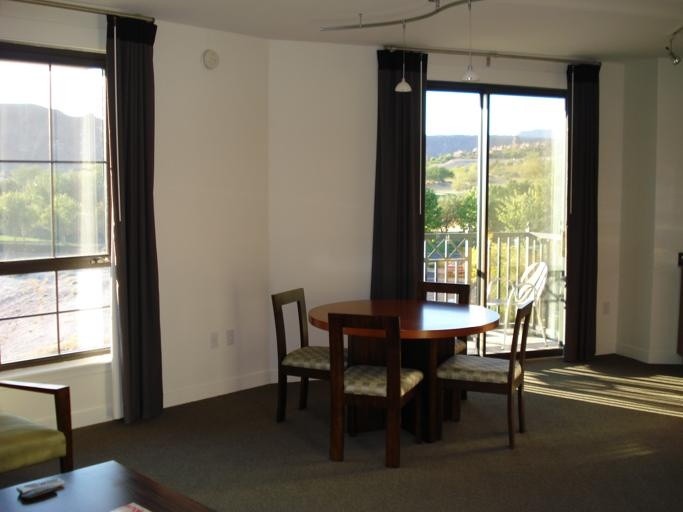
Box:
[325,309,426,468]
[1,377,75,487]
[268,288,353,420]
[406,279,470,422]
[482,257,553,349]
[437,300,539,451]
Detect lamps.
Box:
[393,0,483,92]
[664,30,682,66]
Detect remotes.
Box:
[16,477,65,499]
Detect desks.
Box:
[304,297,502,441]
